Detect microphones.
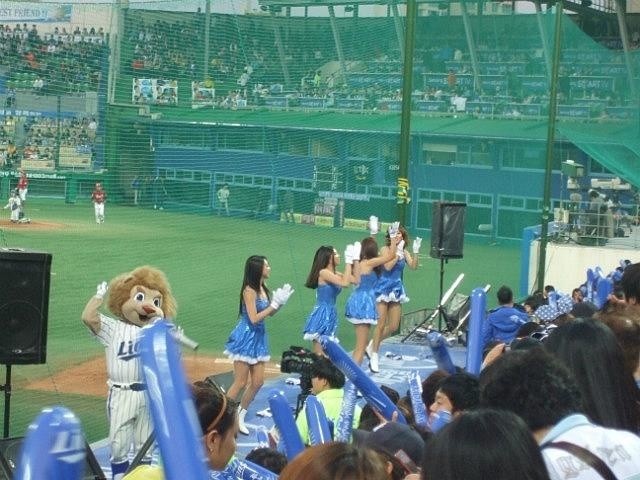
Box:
[171,329,198,350]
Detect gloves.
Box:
[388,221,400,238]
[413,236,422,253]
[396,240,405,260]
[352,241,362,261]
[270,287,289,311]
[279,283,295,305]
[368,215,378,235]
[96,281,109,296]
[344,245,355,264]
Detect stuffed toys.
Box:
[81,266,188,480]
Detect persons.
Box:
[120,384,240,480]
[343,214,401,400]
[15,171,29,204]
[364,227,421,372]
[92,181,108,224]
[129,16,639,117]
[242,261,639,479]
[3,189,33,223]
[222,255,296,438]
[0,16,107,170]
[591,191,607,213]
[303,242,362,356]
[216,183,232,216]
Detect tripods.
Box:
[401,257,452,343]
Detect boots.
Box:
[236,405,249,435]
[364,339,374,358]
[368,351,383,373]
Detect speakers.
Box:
[430,202,466,259]
[0,250,52,365]
[0,434,106,480]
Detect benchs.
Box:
[0,115,101,179]
